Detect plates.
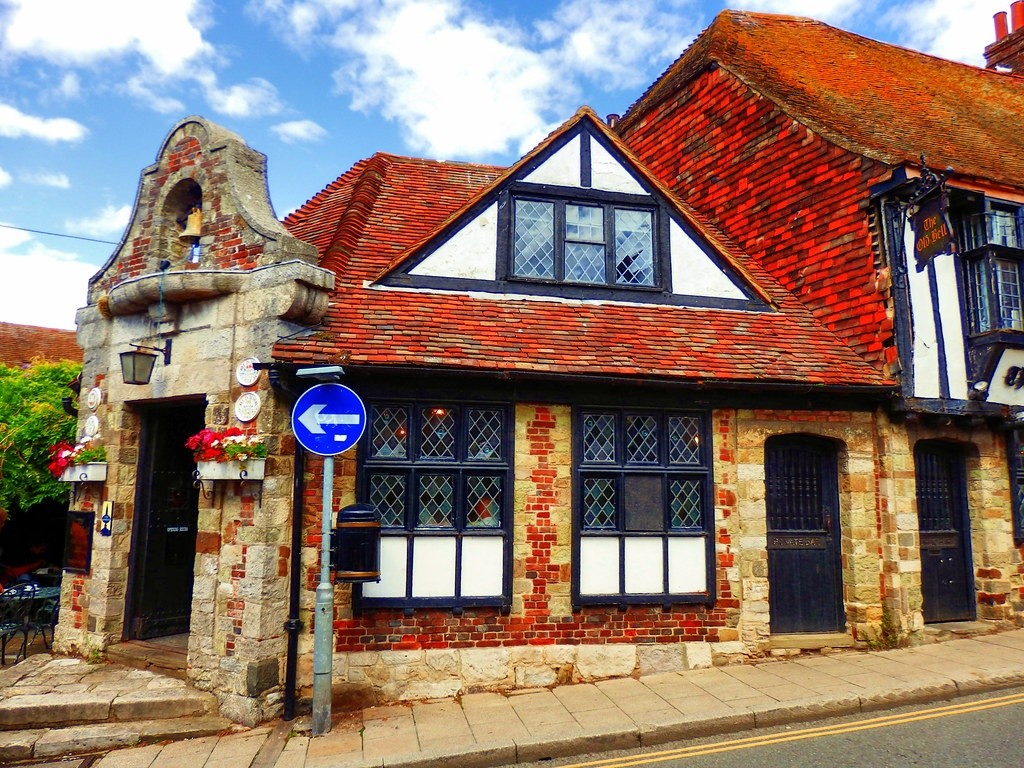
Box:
[0,589,17,595]
[23,589,34,591]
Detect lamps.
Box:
[118,339,172,385]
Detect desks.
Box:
[6,586,60,648]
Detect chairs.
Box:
[0,583,60,665]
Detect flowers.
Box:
[48,441,107,477]
[185,427,267,469]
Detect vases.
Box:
[57,463,107,481]
[196,457,266,485]
